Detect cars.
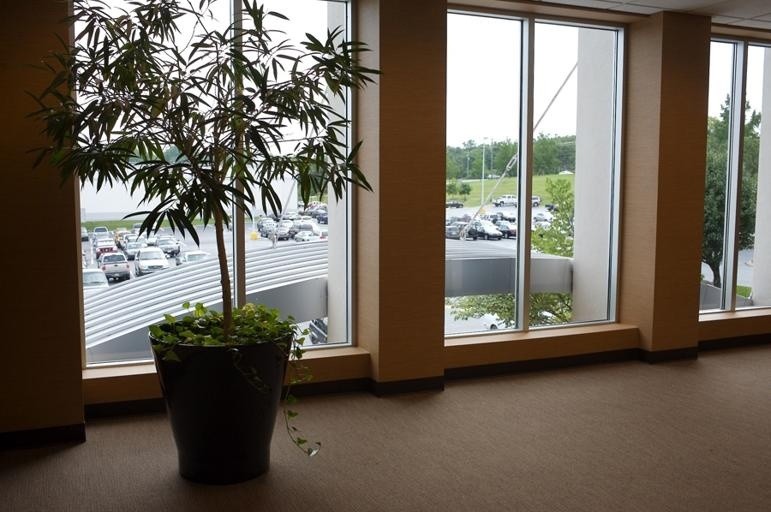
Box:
[308,318,328,344]
[80,223,211,298]
[446,212,553,241]
[446,200,463,208]
[257,201,328,242]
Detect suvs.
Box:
[492,194,516,207]
[514,196,540,207]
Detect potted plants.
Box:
[27,0,384,486]
